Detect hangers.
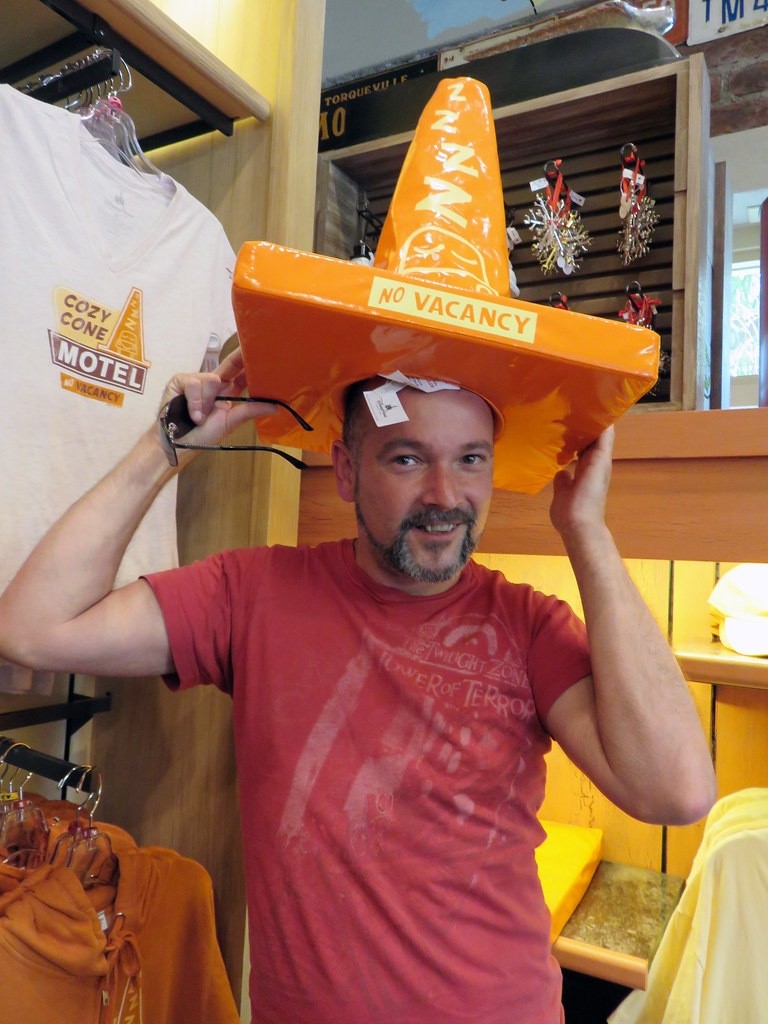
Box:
[0,735,121,889]
[20,48,173,201]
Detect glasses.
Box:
[157,394,314,470]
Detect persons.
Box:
[0,345,715,1023]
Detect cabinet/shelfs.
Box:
[298,0,768,988]
[1,2,330,1023]
